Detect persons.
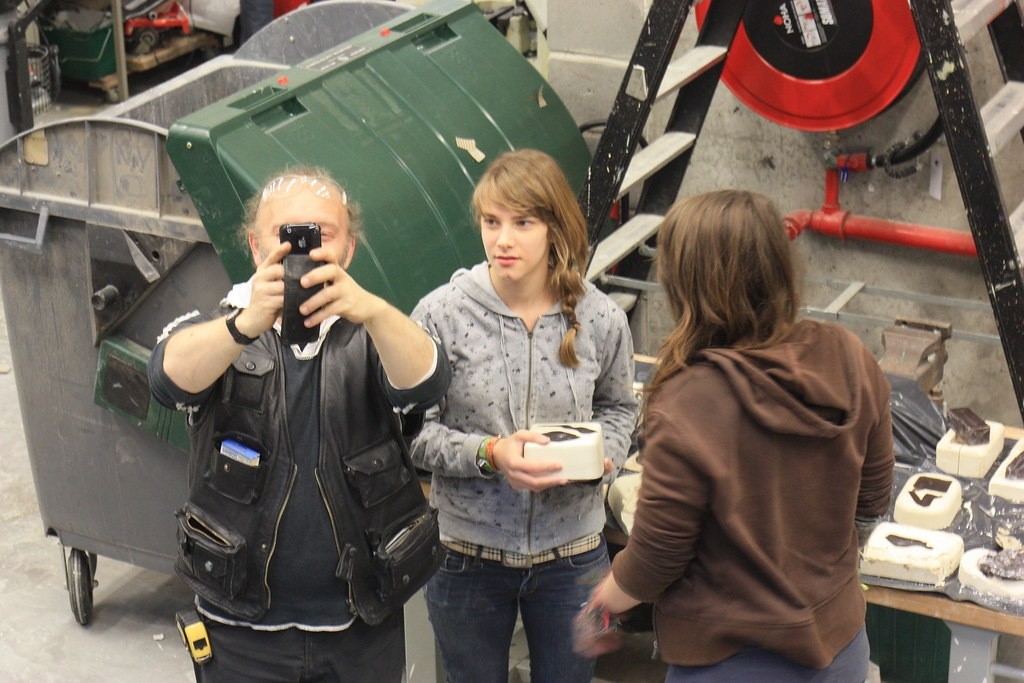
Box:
[571,190,894,683]
[148,168,452,682]
[407,147,638,683]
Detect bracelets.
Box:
[477,436,502,473]
[576,595,627,638]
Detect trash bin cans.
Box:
[1,1,712,578]
[0,44,52,148]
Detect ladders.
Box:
[581,1,1024,433]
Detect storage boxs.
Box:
[39,13,126,80]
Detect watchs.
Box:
[225,306,261,346]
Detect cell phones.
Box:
[279,225,322,265]
